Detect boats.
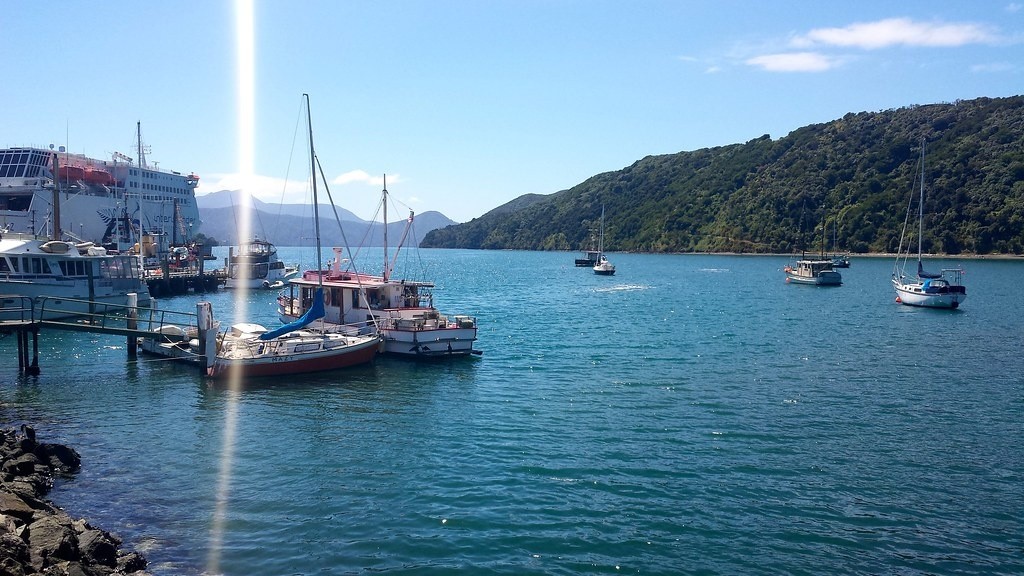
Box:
[223,191,300,289]
[136,94,383,378]
[785,200,850,286]
[0,115,227,320]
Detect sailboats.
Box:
[276,94,485,361]
[892,136,968,308]
[575,204,616,275]
